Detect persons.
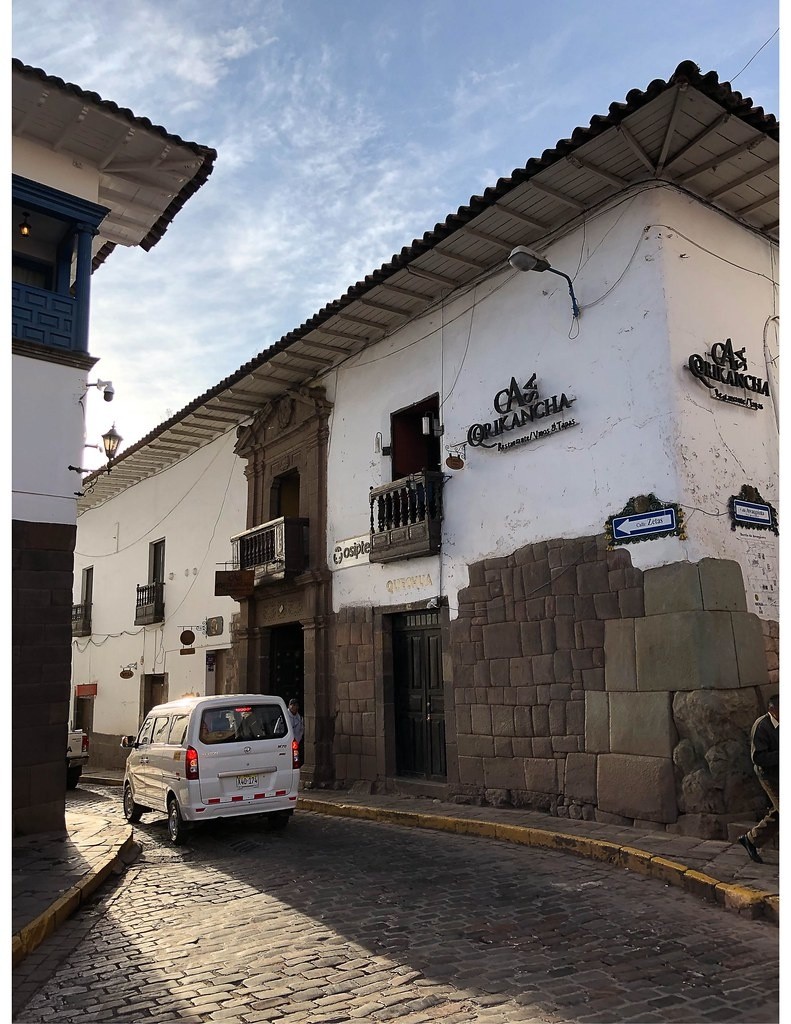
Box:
[737,694,779,863]
[274,699,304,746]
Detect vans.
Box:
[121,693,300,845]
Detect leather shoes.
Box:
[737,834,764,864]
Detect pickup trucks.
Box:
[67,730,90,789]
